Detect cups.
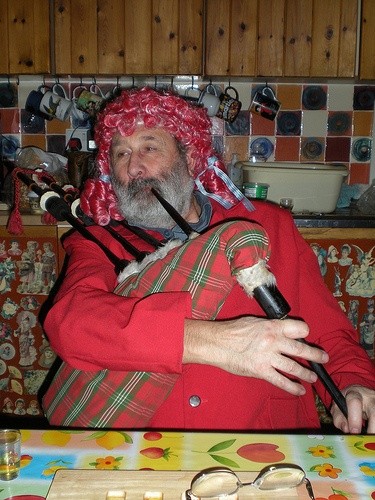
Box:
[280,197,295,211]
[199,82,220,117]
[0,429,22,482]
[75,84,115,116]
[215,86,242,124]
[248,86,283,122]
[40,83,73,121]
[24,85,55,121]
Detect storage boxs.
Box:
[235,159,349,214]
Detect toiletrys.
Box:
[228,152,244,189]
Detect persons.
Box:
[42,87,375,433]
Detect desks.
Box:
[1,429,375,500]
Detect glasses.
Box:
[186,463,315,500]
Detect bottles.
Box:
[63,139,81,158]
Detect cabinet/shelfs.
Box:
[0,0,375,79]
[1,209,375,427]
